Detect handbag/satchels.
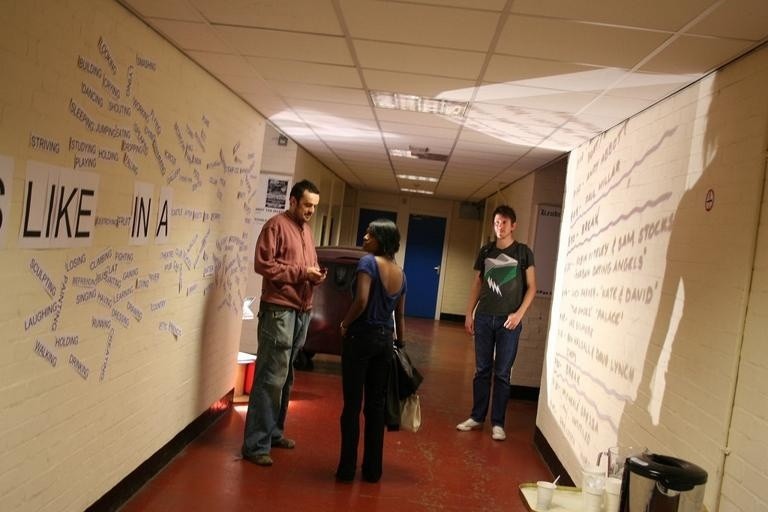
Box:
[398,394,421,433]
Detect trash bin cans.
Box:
[293,245,374,371]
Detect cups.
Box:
[604,477,622,512]
[535,480,558,511]
[581,486,605,512]
[596,444,651,480]
[581,463,606,486]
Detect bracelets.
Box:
[339,320,347,329]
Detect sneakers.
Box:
[256,456,273,464]
[270,440,295,449]
[492,426,507,440]
[457,418,481,433]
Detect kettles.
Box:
[622,453,709,511]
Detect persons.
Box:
[236,178,329,468]
[453,203,536,441]
[332,217,409,484]
